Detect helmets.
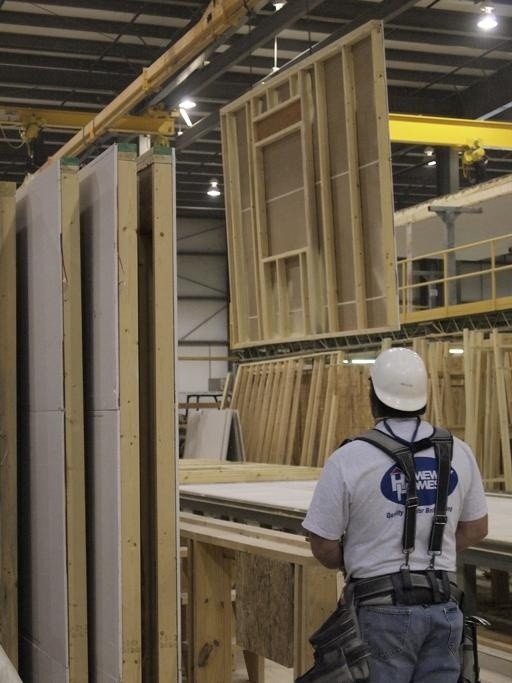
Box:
[369,346,430,411]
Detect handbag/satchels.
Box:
[457,615,492,683]
[294,608,371,683]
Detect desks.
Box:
[176,477,512,682]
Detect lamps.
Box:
[178,98,198,109]
[206,177,221,197]
[475,6,500,32]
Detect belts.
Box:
[354,571,465,604]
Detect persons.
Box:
[301,347,488,683]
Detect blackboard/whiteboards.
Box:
[182,409,233,459]
